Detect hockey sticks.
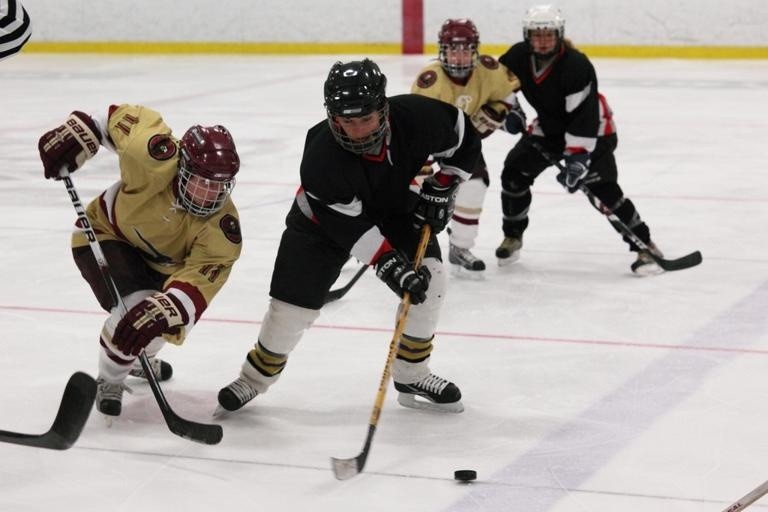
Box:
[520,126,702,271]
[1,371,97,449]
[330,224,432,481]
[58,163,222,445]
[326,263,369,301]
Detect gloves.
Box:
[373,250,431,305]
[557,153,593,193]
[410,181,459,245]
[500,105,527,134]
[111,293,189,357]
[39,111,102,180]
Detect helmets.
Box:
[438,19,479,79]
[178,124,240,218]
[323,56,390,155]
[523,6,565,60]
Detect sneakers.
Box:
[218,378,258,411]
[496,237,522,258]
[449,243,485,270]
[631,241,663,272]
[394,373,461,403]
[129,357,172,381]
[97,376,133,416]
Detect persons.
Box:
[496,4,663,271]
[408,13,526,270]
[218,54,476,413]
[37,99,243,417]
